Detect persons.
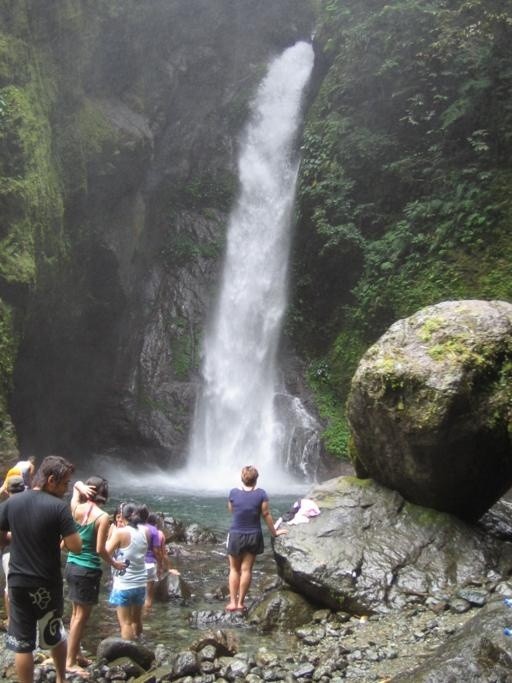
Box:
[0,454,83,683]
[223,464,290,611]
[66,474,127,680]
[103,500,173,641]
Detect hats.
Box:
[7,476,26,493]
